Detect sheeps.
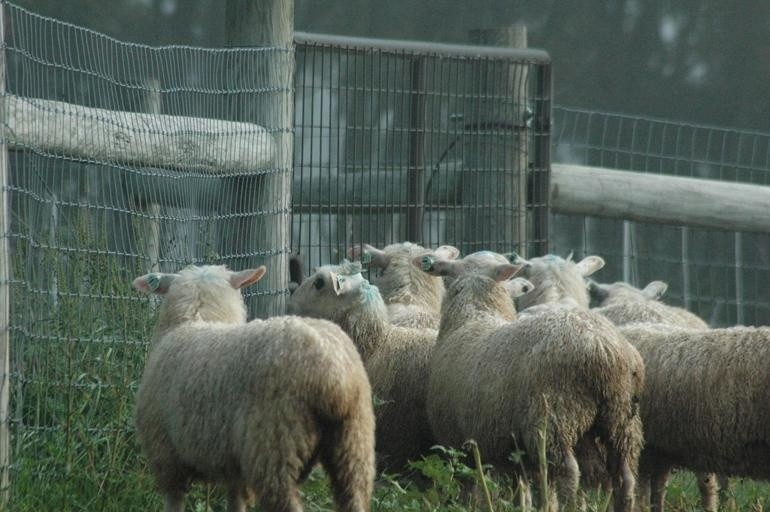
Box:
[129,262,379,512]
[287,237,770,512]
